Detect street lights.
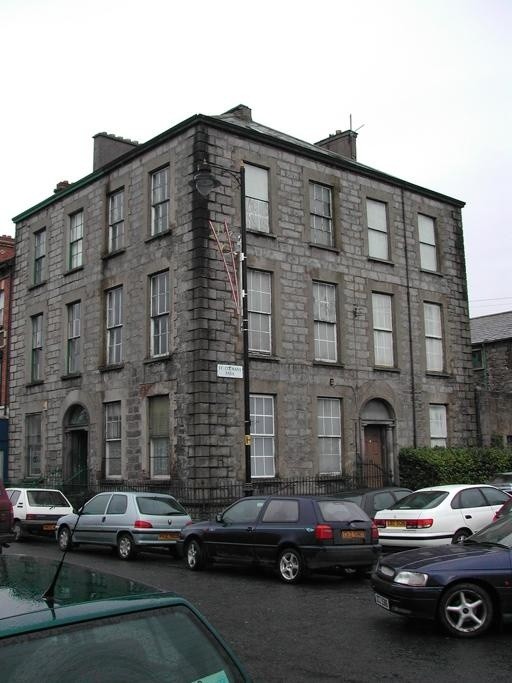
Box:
[187,161,252,496]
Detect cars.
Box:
[5,487,74,541]
[337,487,413,522]
[373,514,512,637]
[55,492,193,560]
[176,494,380,585]
[374,483,511,549]
[0,552,250,683]
